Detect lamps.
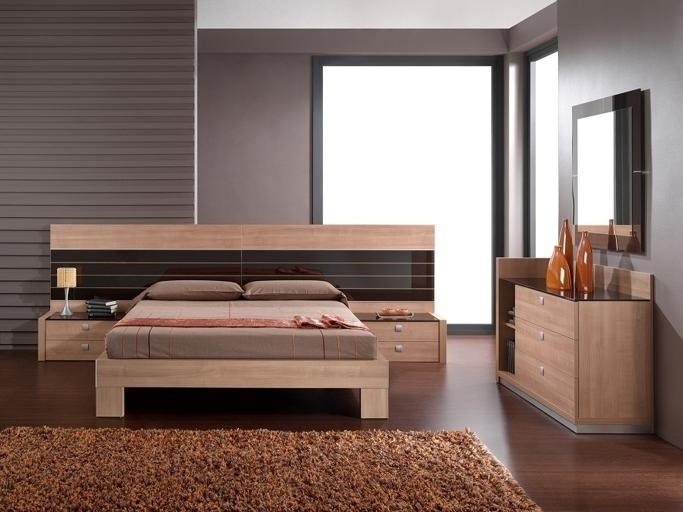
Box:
[55,267,78,315]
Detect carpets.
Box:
[0,426,547,511]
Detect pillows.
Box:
[143,278,244,301]
[241,280,346,301]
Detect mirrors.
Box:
[572,88,645,256]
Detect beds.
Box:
[94,287,390,418]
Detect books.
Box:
[83,297,119,316]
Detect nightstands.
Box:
[37,311,125,363]
[353,312,447,366]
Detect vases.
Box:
[544,218,595,297]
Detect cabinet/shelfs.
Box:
[496,273,654,435]
